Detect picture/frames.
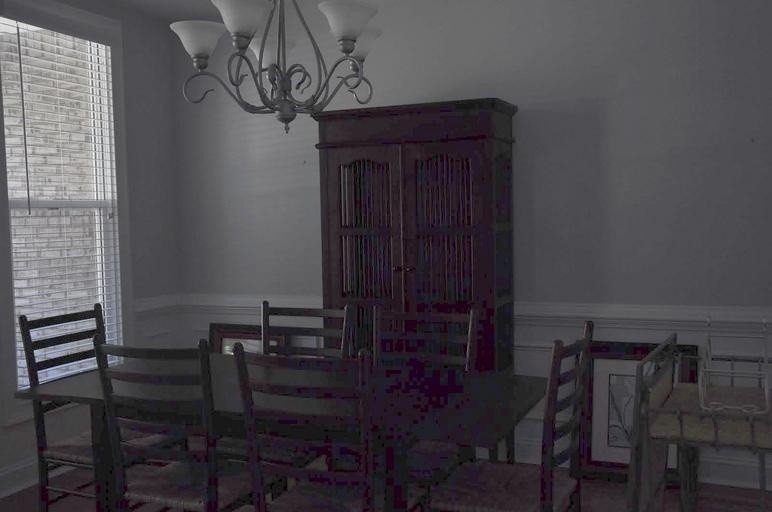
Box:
[210,322,289,356]
[570,340,699,488]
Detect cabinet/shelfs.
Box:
[313,99,513,374]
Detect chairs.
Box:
[20,304,189,512]
[422,320,594,512]
[95,331,289,511]
[263,301,357,358]
[232,341,397,511]
[369,303,481,371]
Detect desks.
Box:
[15,354,550,487]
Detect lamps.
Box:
[170,0,373,134]
